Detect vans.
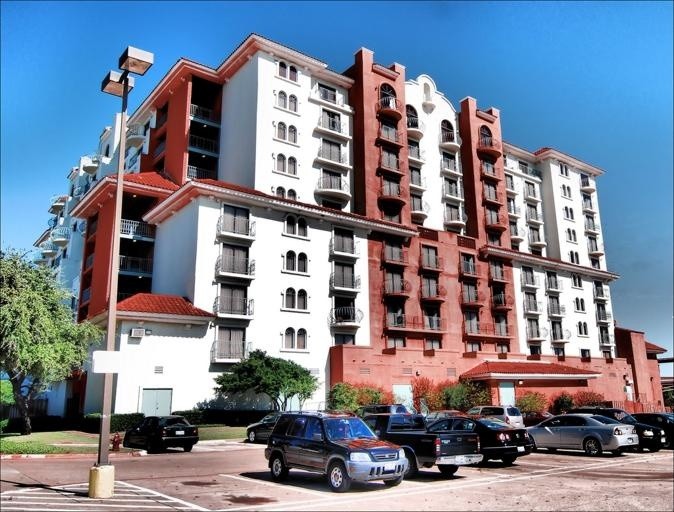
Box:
[354,404,408,420]
[466,406,526,429]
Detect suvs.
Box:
[565,407,667,453]
[265,411,409,493]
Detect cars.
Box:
[425,410,466,426]
[520,410,555,427]
[471,416,533,467]
[124,415,198,455]
[525,413,639,457]
[620,412,674,450]
[246,412,293,444]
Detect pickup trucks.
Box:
[361,413,483,480]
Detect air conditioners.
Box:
[130,328,146,337]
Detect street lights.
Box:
[87,43,155,498]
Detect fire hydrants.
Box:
[109,431,122,452]
